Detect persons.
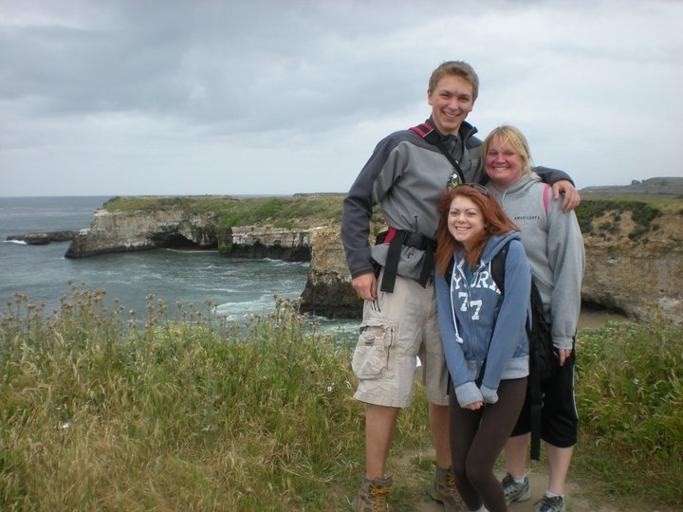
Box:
[464,126,585,512]
[339,60,584,512]
[430,180,540,511]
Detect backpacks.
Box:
[444,242,553,406]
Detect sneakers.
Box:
[533,495,564,512]
[352,473,393,512]
[500,472,531,507]
[427,465,471,512]
